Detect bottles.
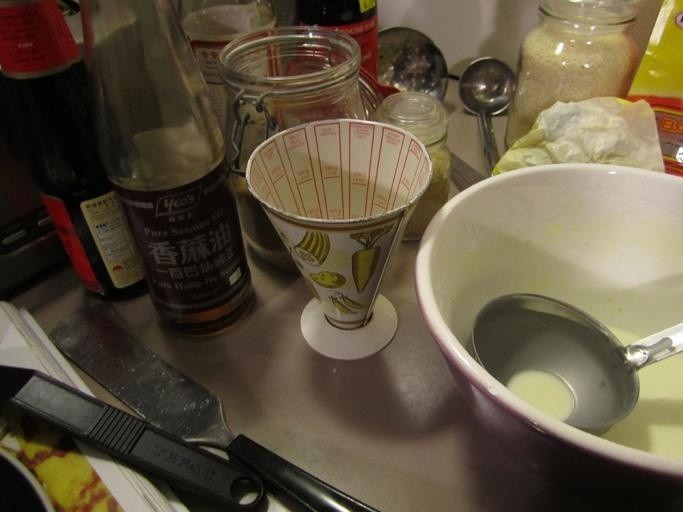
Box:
[506,0,638,152]
[0,0,454,337]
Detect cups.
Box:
[246,118,432,360]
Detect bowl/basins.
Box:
[414,164,683,485]
